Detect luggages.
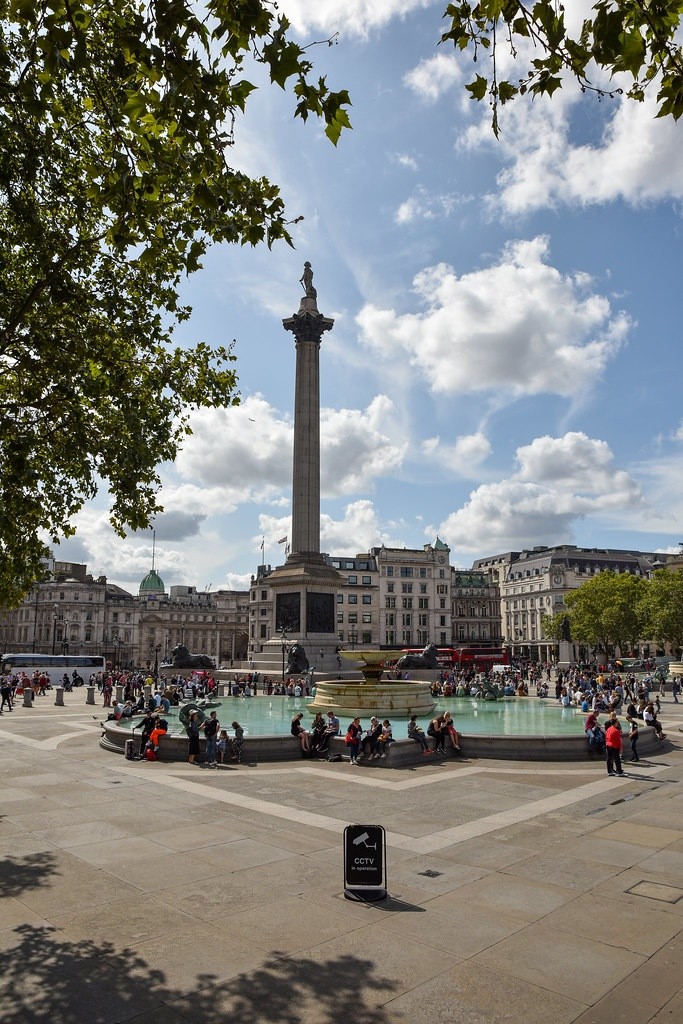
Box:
[124,727,135,760]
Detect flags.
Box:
[278,537,288,544]
[260,540,264,549]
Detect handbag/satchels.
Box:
[327,753,342,762]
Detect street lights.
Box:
[154,645,161,690]
[280,632,288,695]
[63,617,69,656]
[350,623,355,651]
[113,635,119,686]
[52,611,59,655]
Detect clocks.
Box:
[554,576,562,584]
[438,556,446,564]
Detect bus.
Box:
[0,653,106,687]
[401,648,460,669]
[453,647,508,673]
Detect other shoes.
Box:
[205,760,217,765]
[358,751,366,757]
[374,753,380,759]
[660,736,668,742]
[145,740,152,746]
[350,759,358,765]
[454,743,460,750]
[433,747,440,755]
[368,754,374,760]
[608,771,629,776]
[422,748,434,756]
[439,746,447,754]
[381,753,387,759]
[154,745,159,751]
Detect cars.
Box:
[627,660,647,672]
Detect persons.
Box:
[1,650,683,777]
